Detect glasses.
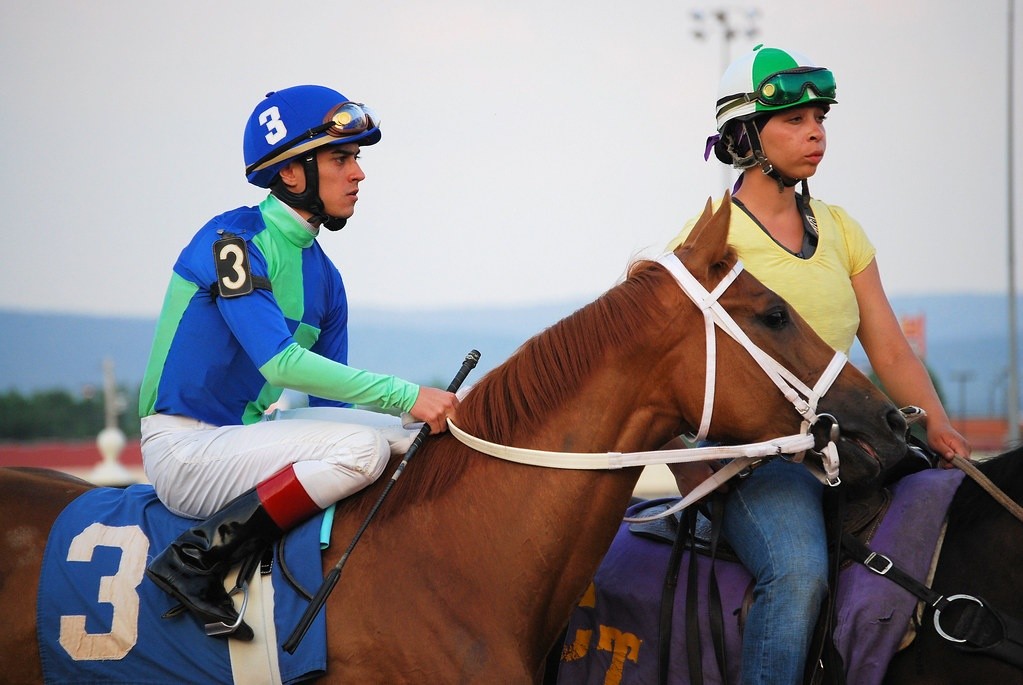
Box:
[755,67,837,107]
[321,100,382,138]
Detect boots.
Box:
[143,463,325,641]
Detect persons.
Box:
[136,84,462,643]
[661,43,973,685]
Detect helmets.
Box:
[243,85,382,188]
[703,45,838,164]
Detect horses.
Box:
[554,437,1023,685]
[2,190,909,683]
